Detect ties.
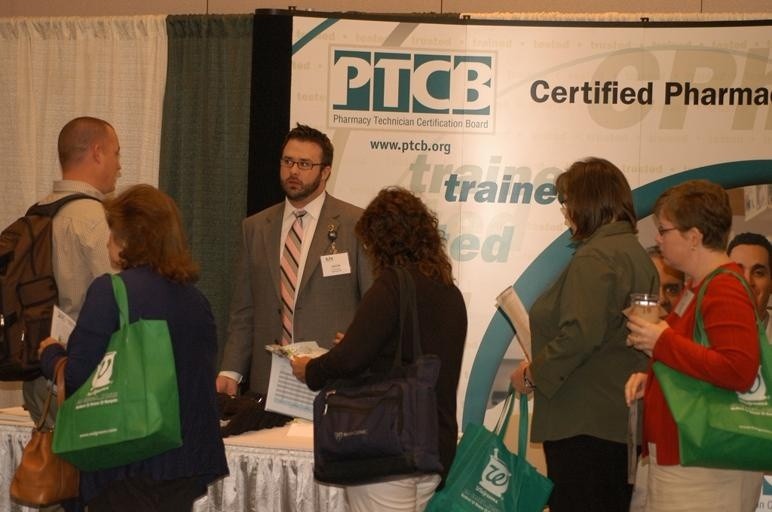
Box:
[279,209,307,346]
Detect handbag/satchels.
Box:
[52,320,184,472]
[650,332,772,472]
[10,428,81,507]
[423,423,554,512]
[314,354,445,484]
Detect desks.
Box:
[0,405,349,512]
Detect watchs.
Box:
[523,368,536,389]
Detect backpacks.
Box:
[0,193,105,382]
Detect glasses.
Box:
[657,225,676,237]
[280,158,324,170]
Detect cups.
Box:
[629,292,661,350]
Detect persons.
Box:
[38,183,226,512]
[627,178,762,512]
[510,160,658,512]
[728,232,772,349]
[24,117,118,426]
[291,187,466,511]
[644,247,684,316]
[215,122,374,399]
